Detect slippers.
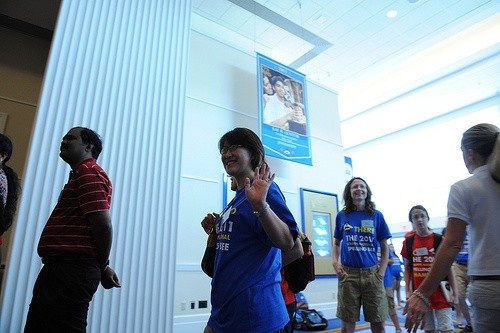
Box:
[458,323,465,328]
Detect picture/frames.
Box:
[300,187,339,278]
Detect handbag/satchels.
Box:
[201,225,217,278]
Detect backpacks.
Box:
[302,309,328,331]
[284,222,315,294]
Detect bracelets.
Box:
[412,289,430,307]
[253,204,269,216]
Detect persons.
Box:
[23,126,122,333]
[441,226,448,235]
[402,123,500,333]
[201,126,300,333]
[332,177,392,333]
[263,75,307,131]
[201,160,304,333]
[377,242,402,333]
[388,243,405,307]
[0,134,21,247]
[400,204,454,333]
[450,224,472,333]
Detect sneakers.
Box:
[460,325,473,333]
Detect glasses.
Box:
[221,144,242,154]
